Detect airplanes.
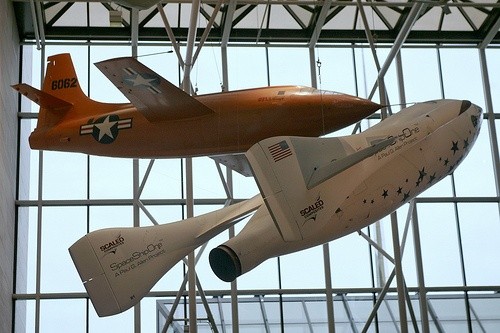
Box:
[66,98,484,316]
[9,53,384,159]
